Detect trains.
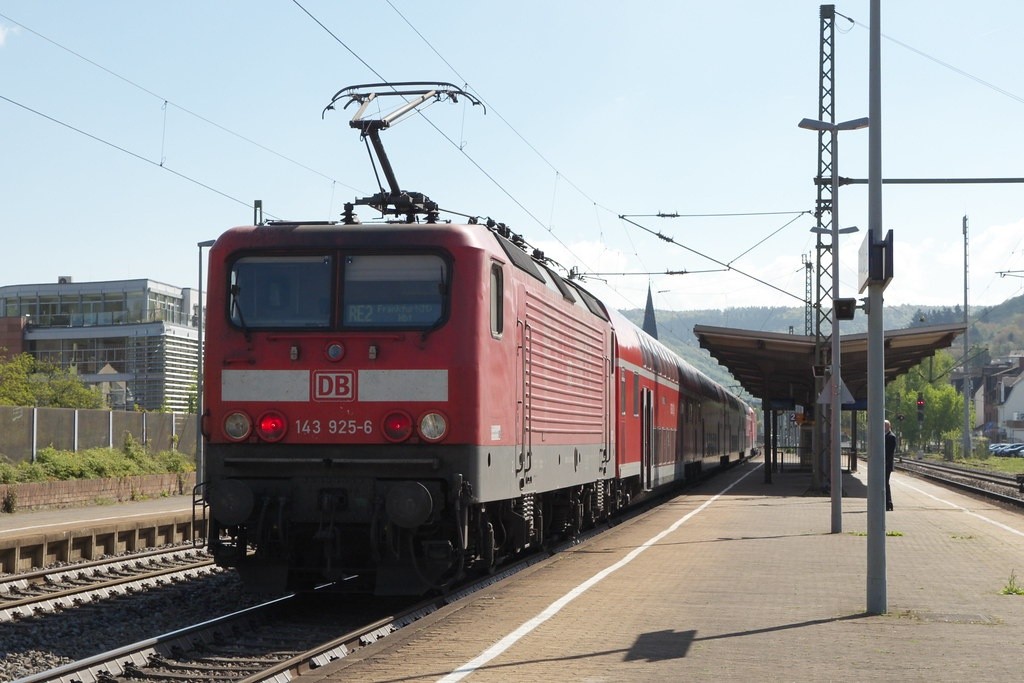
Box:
[191,80,761,600]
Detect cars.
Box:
[988,442,1024,458]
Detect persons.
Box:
[885,420,896,511]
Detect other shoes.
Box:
[886,502,893,511]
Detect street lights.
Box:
[798,117,868,533]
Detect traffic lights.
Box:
[917,397,924,418]
